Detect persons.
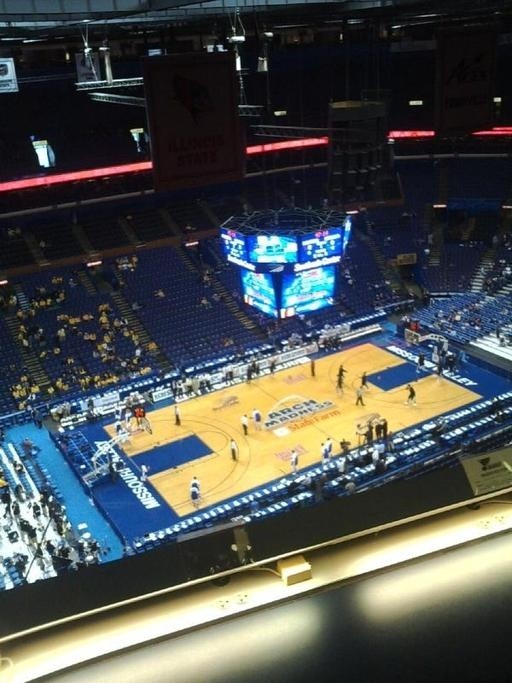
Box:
[0,169,511,585]
